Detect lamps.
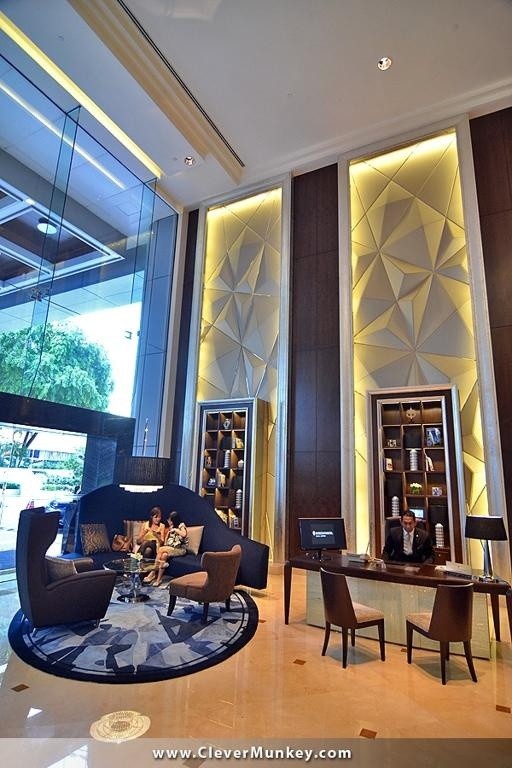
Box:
[88,710,152,745]
[464,514,509,576]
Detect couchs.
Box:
[54,481,268,589]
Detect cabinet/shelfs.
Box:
[191,396,268,543]
[367,383,466,565]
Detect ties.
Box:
[403,533,411,556]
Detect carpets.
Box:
[7,574,260,684]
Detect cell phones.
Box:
[416,562,421,567]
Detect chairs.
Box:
[318,567,386,668]
[405,581,480,684]
[167,543,242,621]
[15,506,117,636]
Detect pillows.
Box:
[122,518,149,553]
[78,521,112,556]
[185,524,204,556]
[43,554,79,583]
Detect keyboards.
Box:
[385,560,407,565]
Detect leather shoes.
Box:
[152,579,162,587]
[143,575,156,583]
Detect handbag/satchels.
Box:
[111,534,131,552]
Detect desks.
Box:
[282,551,512,644]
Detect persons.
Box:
[383,510,436,564]
[143,510,189,587]
[136,507,164,559]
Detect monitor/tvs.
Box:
[298,518,347,561]
